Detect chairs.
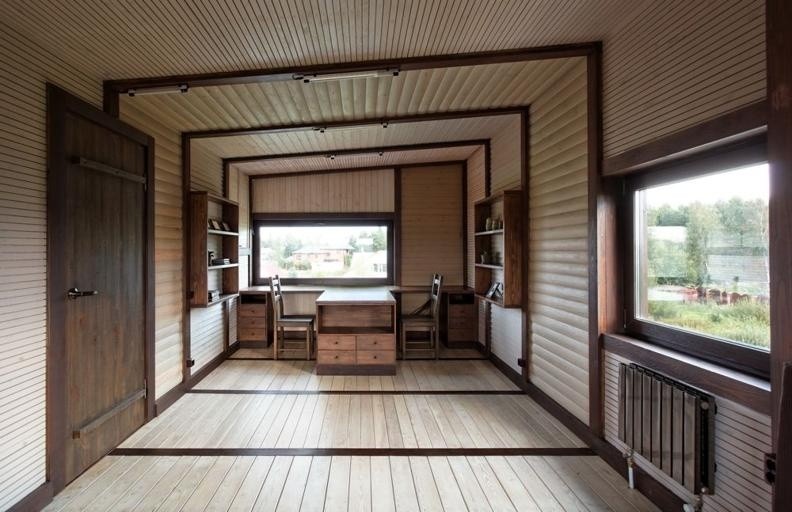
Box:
[267,273,443,361]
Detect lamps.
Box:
[303,67,400,159]
[127,83,189,98]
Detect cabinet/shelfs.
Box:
[238,286,476,376]
[189,190,240,307]
[473,188,521,309]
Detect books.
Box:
[212,257,231,265]
[208,290,221,302]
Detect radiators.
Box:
[617,362,716,512]
